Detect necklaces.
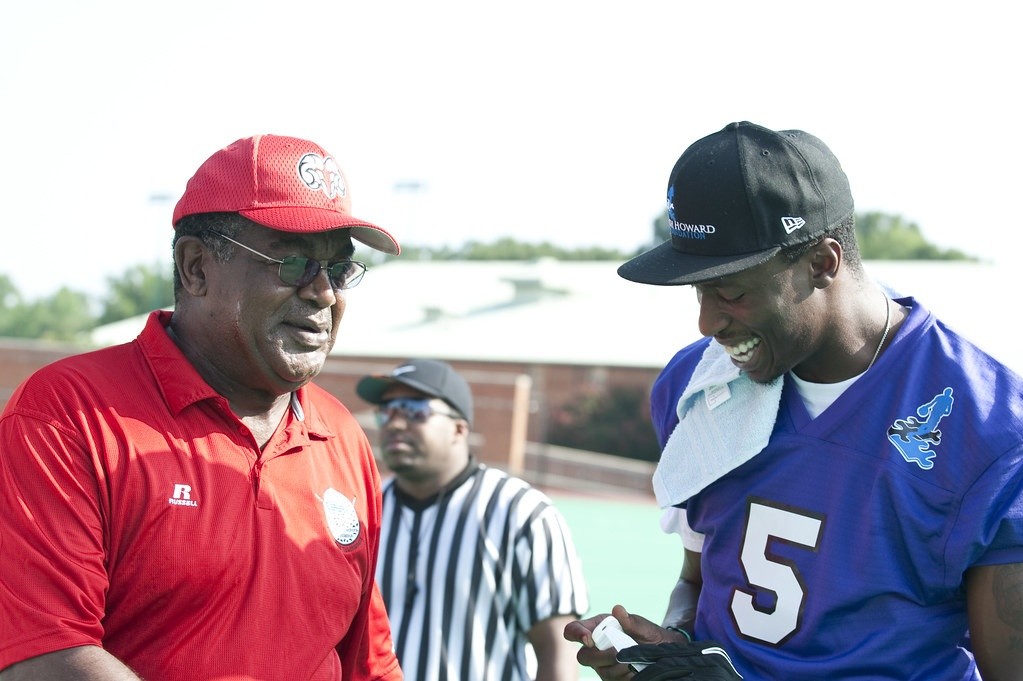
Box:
[866,289,891,371]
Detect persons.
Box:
[562,115,1023,681]
[3,132,410,681]
[355,358,590,680]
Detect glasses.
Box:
[374,398,467,424]
[206,224,368,289]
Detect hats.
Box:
[174,134,399,254]
[614,121,853,286]
[358,360,477,425]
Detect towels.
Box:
[651,332,785,513]
